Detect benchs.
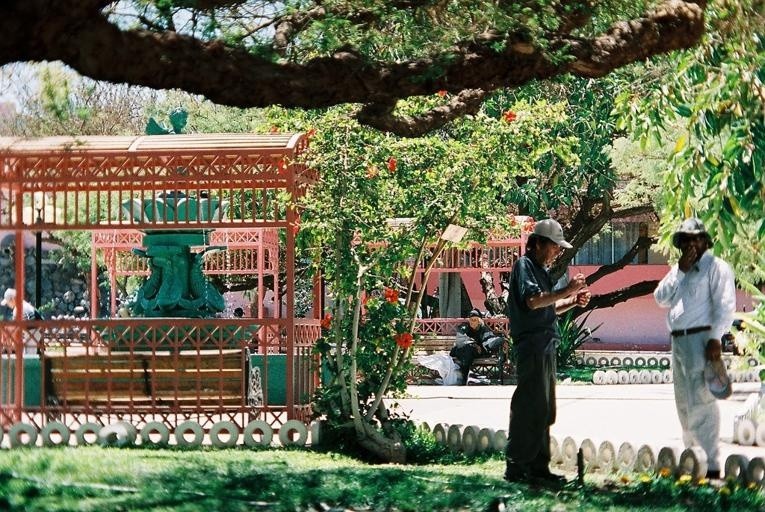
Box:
[407,339,504,384]
[45,348,249,435]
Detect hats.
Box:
[469,310,480,316]
[534,218,574,250]
[704,358,733,400]
[234,308,244,317]
[672,217,714,249]
[1,287,17,306]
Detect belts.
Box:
[671,326,711,337]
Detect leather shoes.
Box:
[503,463,568,490]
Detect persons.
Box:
[449,306,504,386]
[0,288,45,356]
[498,215,593,491]
[651,215,737,481]
